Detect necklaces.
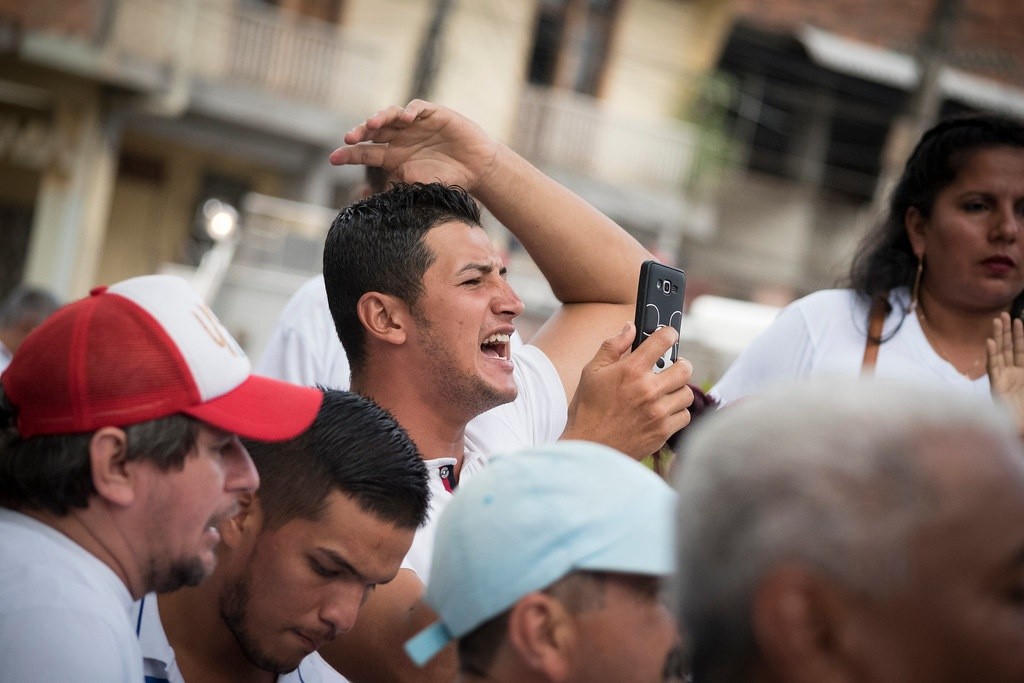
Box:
[917,306,999,379]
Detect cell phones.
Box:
[631,260,686,375]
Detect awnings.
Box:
[722,18,1024,114]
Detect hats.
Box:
[1,273,323,444]
[405,440,679,668]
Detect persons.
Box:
[404,441,679,683]
[129,385,434,683]
[0,276,322,683]
[664,113,1024,683]
[317,98,694,683]
[269,163,524,393]
[673,383,1024,682]
